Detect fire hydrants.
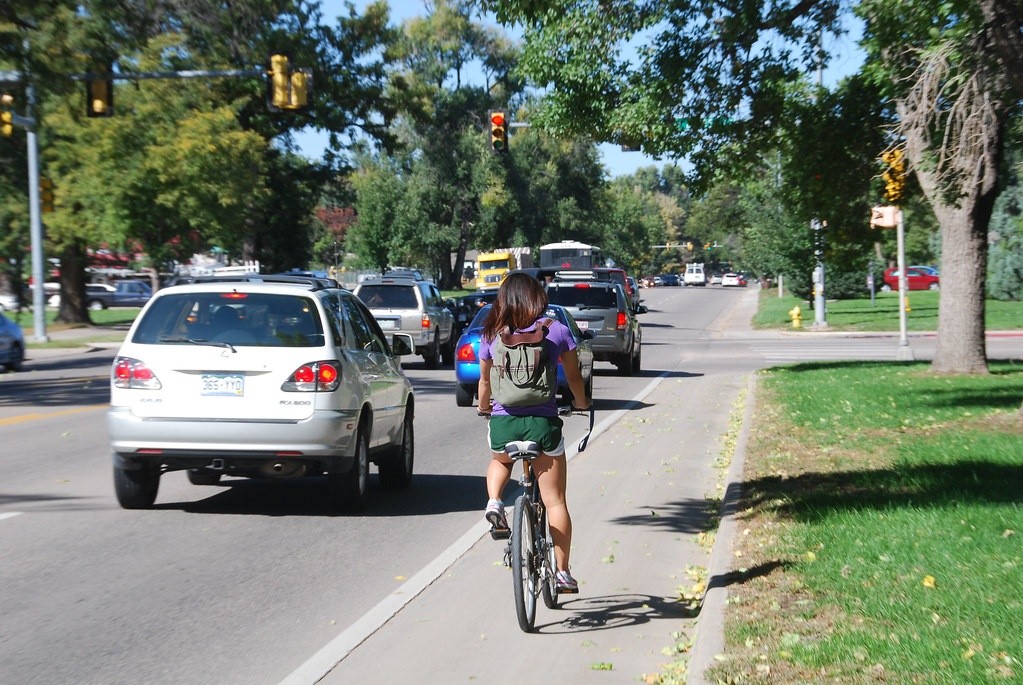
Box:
[788,306,803,328]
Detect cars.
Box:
[453,305,597,407]
[879,267,939,292]
[442,267,643,348]
[892,265,939,277]
[0,258,325,371]
[640,262,748,287]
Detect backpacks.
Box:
[490,316,556,408]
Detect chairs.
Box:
[214,306,238,325]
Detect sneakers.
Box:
[554,569,578,589]
[485,499,509,532]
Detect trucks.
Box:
[474,245,534,293]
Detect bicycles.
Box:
[475,404,597,634]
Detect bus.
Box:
[540,238,601,272]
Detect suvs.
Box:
[106,268,417,511]
[544,277,648,376]
[351,266,457,367]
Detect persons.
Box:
[476,273,593,589]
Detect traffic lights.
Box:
[705,243,712,251]
[489,113,506,152]
[0,91,15,139]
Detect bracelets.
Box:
[479,406,490,411]
